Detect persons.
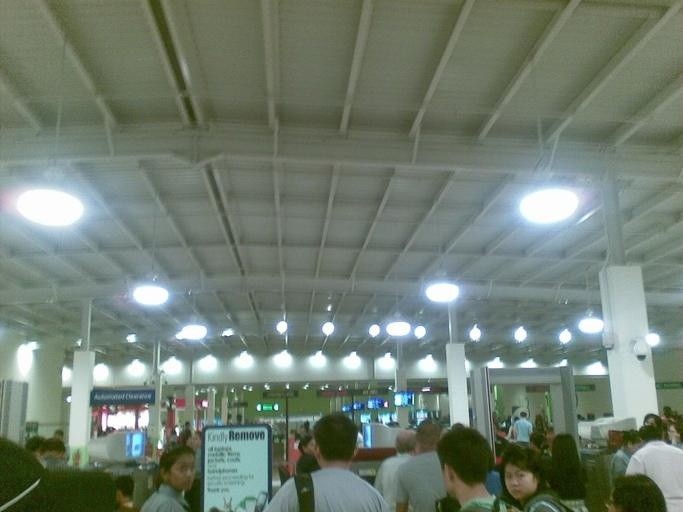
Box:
[0,406,683,512]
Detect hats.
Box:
[0,436,116,512]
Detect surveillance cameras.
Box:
[634,342,649,361]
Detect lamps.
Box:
[16,29,83,228]
[577,263,605,334]
[132,183,170,306]
[386,272,411,336]
[520,58,579,227]
[425,197,460,305]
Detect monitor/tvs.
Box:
[361,422,400,449]
[577,417,637,447]
[87,430,145,467]
